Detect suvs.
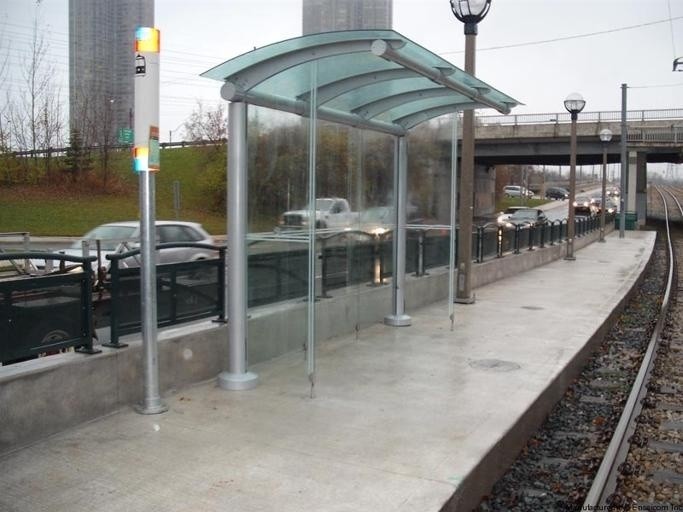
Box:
[545,187,570,201]
[502,186,534,199]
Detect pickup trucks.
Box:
[277,198,361,230]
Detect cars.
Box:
[572,187,621,216]
[29,220,219,293]
[497,206,549,229]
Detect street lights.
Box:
[450,0,492,304]
[598,128,613,242]
[563,92,586,260]
